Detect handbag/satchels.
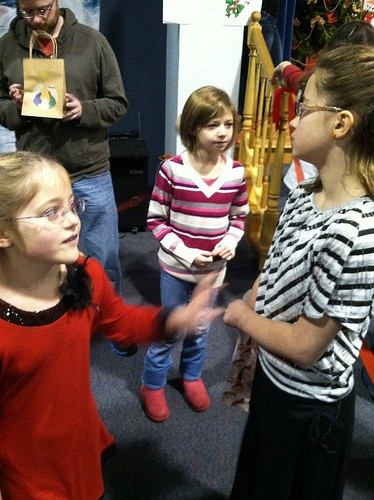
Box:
[20,29,68,120]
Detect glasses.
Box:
[294,100,355,137]
[1,197,86,225]
[18,0,54,19]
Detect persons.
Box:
[223,43,374,500]
[0,151,229,500]
[270,22,374,215]
[140,85,249,421]
[0,0,137,356]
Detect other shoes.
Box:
[140,383,171,422]
[182,378,210,412]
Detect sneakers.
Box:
[110,339,138,356]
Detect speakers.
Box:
[110,132,148,233]
